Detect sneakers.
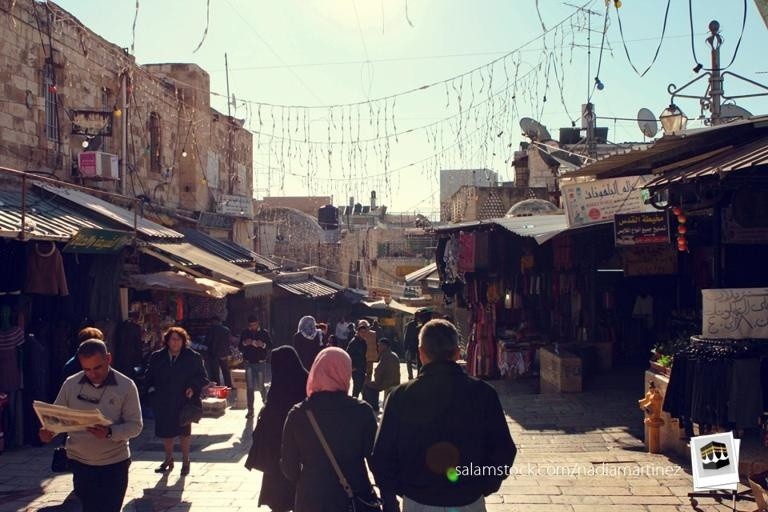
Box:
[245,410,254,419]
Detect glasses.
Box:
[76,379,107,404]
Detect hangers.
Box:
[674,337,753,363]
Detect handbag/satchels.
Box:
[50,447,73,474]
[175,398,203,428]
[339,478,384,512]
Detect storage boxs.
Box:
[202,396,228,419]
[229,367,247,388]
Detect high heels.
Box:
[154,457,174,472]
[180,458,190,476]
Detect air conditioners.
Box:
[77,149,120,178]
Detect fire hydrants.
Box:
[638,380,666,456]
